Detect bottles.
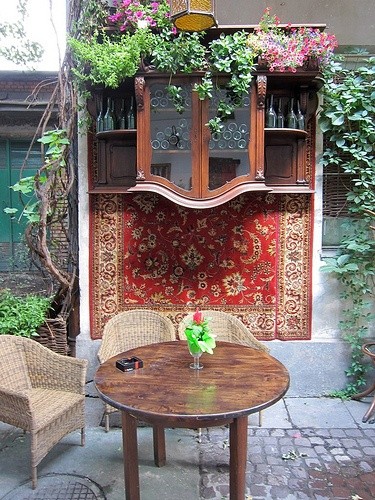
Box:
[264,95,278,128]
[103,97,113,131]
[277,98,285,127]
[117,99,127,129]
[209,122,250,150]
[151,118,193,149]
[110,100,118,129]
[297,100,305,130]
[286,98,297,128]
[150,83,193,109]
[95,111,103,132]
[208,85,251,108]
[127,95,135,129]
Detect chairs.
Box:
[97,308,270,443]
[0,334,89,490]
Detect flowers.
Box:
[183,306,217,355]
[249,3,339,73]
[109,0,177,38]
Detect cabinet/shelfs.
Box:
[87,70,325,209]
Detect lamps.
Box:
[168,0,218,35]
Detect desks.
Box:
[94,340,291,500]
